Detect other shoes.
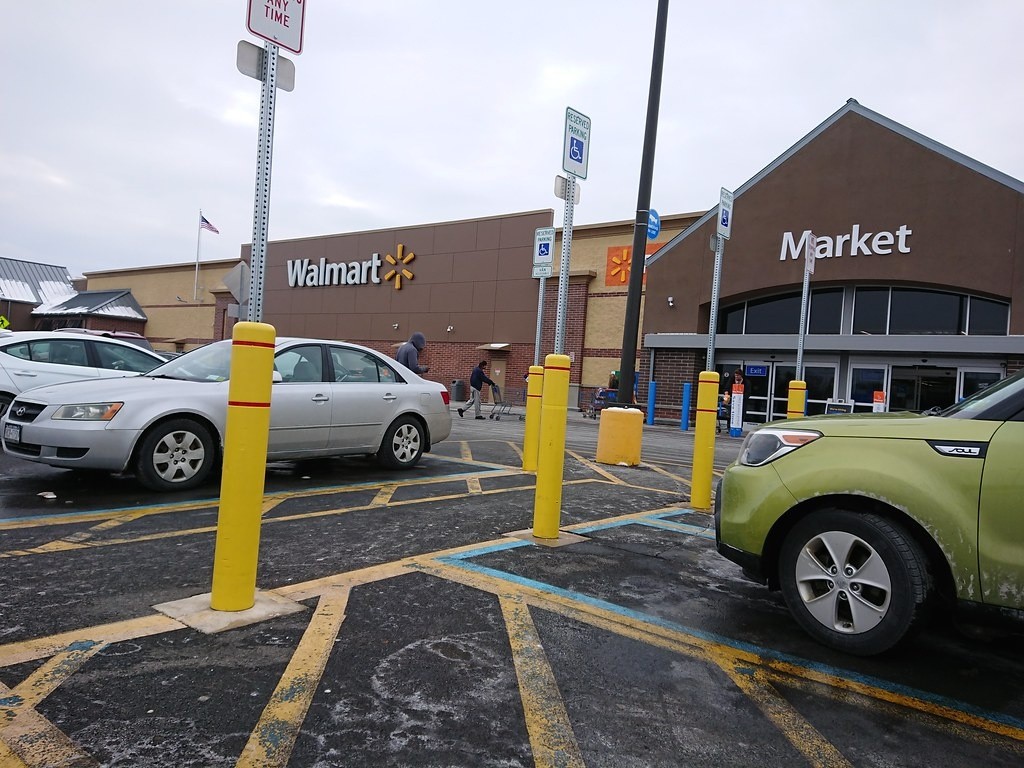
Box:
[475,416,486,419]
[458,408,464,417]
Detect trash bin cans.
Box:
[451,379,466,401]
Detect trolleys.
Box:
[488,383,528,421]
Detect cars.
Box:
[0,327,227,422]
[713,365,1024,660]
[0,335,453,493]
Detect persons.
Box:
[457,361,496,420]
[719,368,750,434]
[608,375,619,389]
[395,332,430,382]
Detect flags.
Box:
[200,215,219,234]
